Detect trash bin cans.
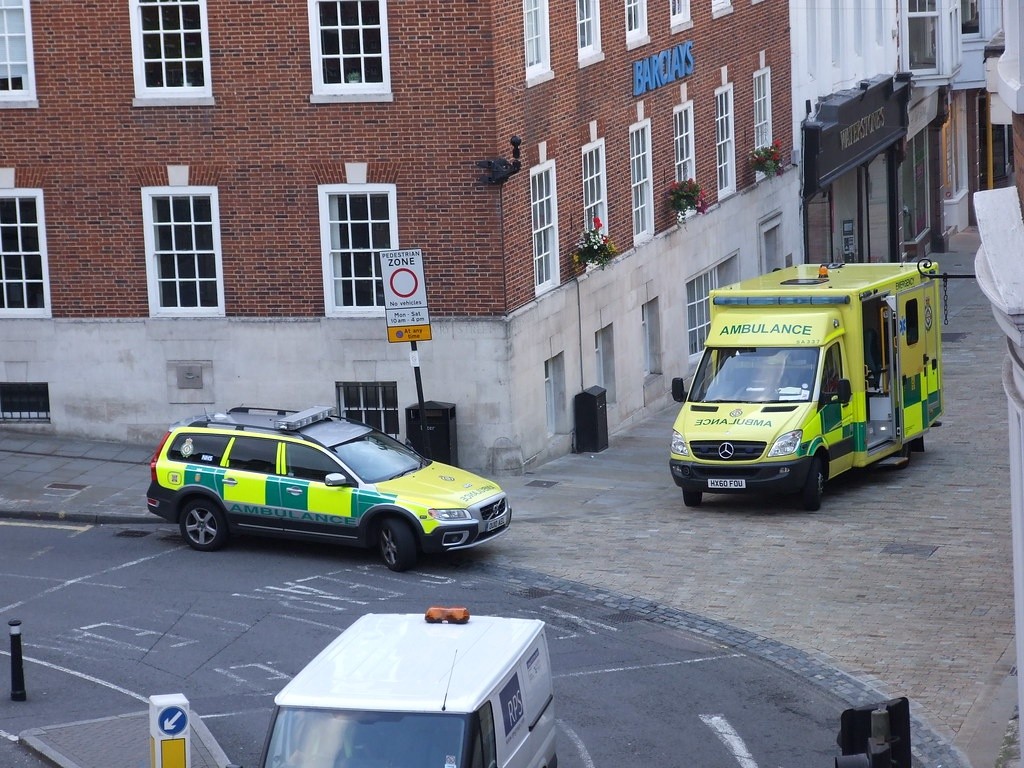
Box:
[574,385,609,453]
[405,401,458,468]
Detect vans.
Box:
[227,608,557,768]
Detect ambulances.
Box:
[669,262,943,511]
[147,406,512,571]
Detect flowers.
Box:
[666,178,709,232]
[568,212,622,273]
[749,137,785,184]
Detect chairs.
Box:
[730,350,761,396]
[772,352,813,389]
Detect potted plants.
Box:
[347,71,361,83]
[180,73,195,86]
